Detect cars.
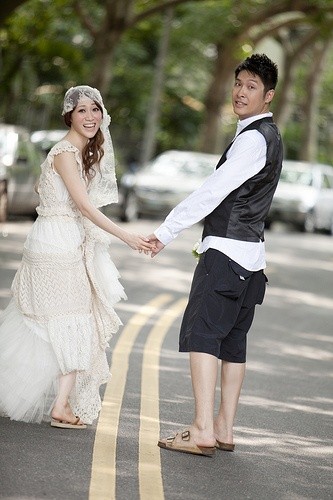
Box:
[119,147,223,220]
[260,158,333,232]
[0,123,48,222]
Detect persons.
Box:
[0,85,154,430]
[145,53,282,457]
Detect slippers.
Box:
[158,428,235,456]
[50,416,87,430]
